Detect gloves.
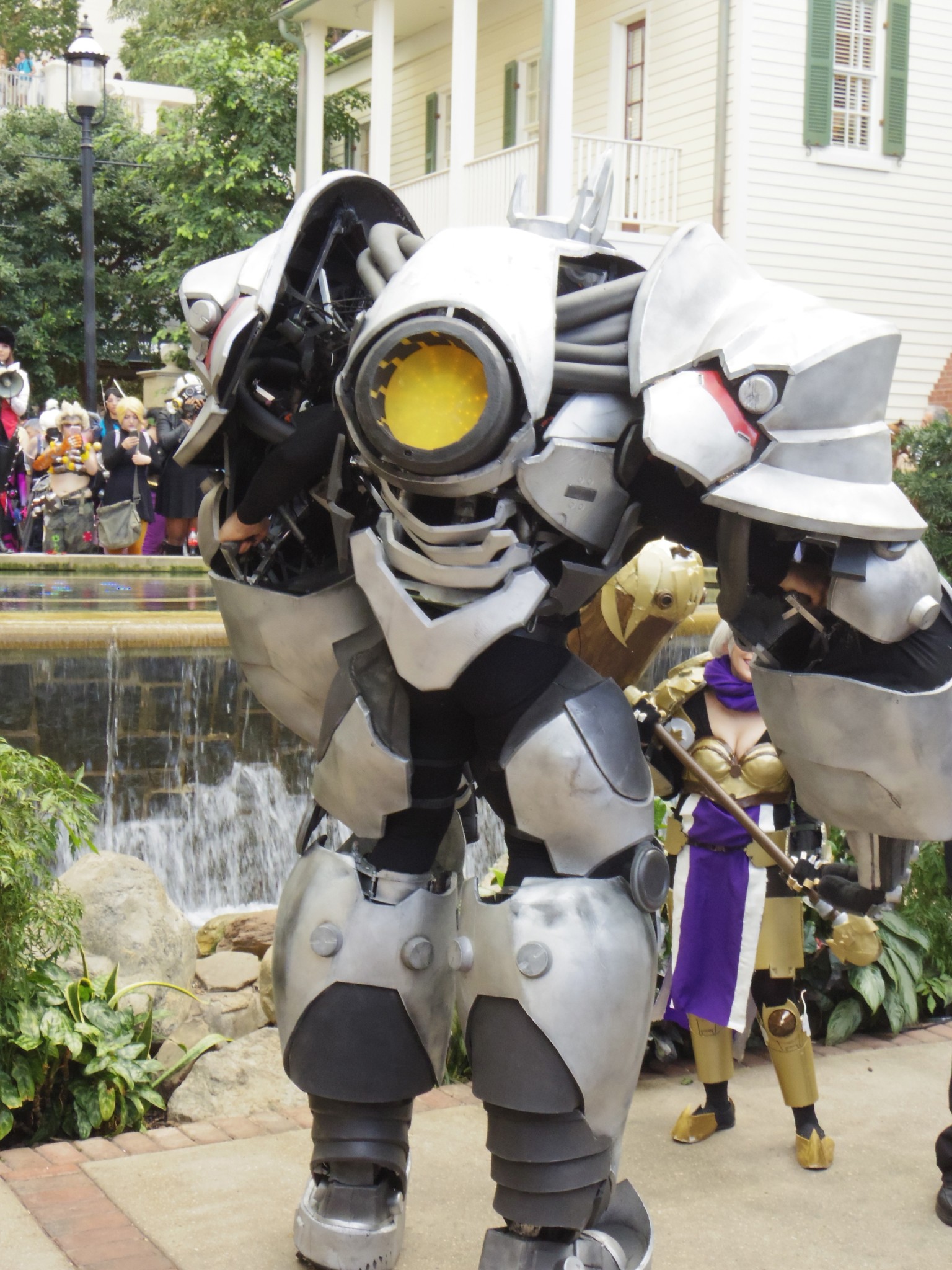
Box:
[32,433,82,471]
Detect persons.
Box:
[0,326,224,556]
[622,619,881,1170]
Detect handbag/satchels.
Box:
[96,499,141,550]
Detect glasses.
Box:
[60,421,82,429]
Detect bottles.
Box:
[187,527,200,556]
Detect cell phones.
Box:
[128,429,138,437]
[70,426,81,437]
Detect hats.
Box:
[0,326,15,352]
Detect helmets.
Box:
[170,373,208,410]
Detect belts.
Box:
[58,497,93,506]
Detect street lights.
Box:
[61,12,111,414]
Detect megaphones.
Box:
[0,367,24,399]
